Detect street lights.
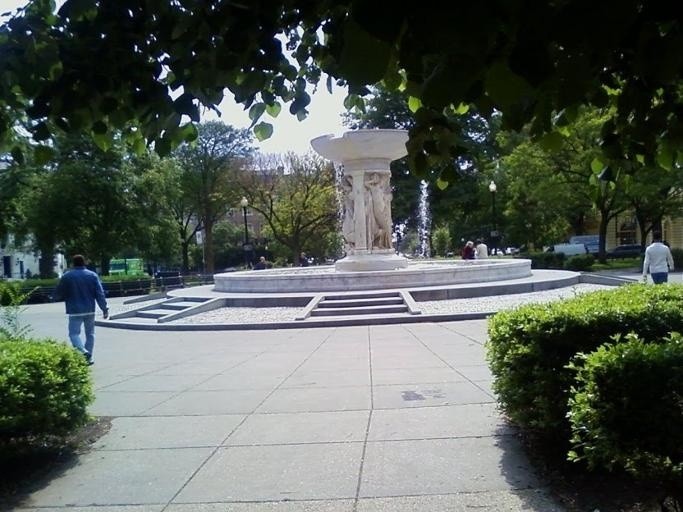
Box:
[239,196,248,271]
[489,181,496,255]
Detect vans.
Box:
[544,234,649,259]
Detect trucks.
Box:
[108,257,144,277]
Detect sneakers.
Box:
[84,351,94,364]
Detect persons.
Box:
[55,254,108,366]
[461,241,474,259]
[254,255,269,269]
[300,252,308,267]
[474,238,488,258]
[642,229,675,284]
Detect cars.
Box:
[491,247,520,256]
[155,270,185,288]
